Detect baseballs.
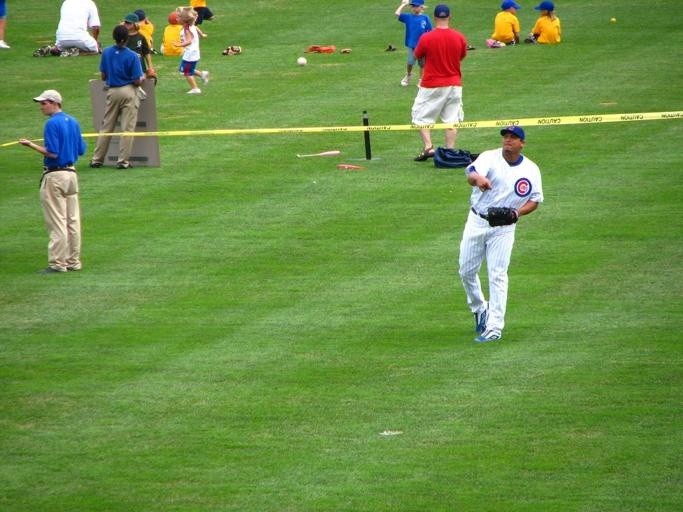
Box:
[298,57,307,65]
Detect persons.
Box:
[52,0,102,59]
[457,124,544,343]
[410,3,469,162]
[172,6,208,94]
[159,11,183,56]
[0,1,12,50]
[16,88,86,275]
[88,25,148,170]
[484,0,523,45]
[189,0,214,27]
[124,13,156,80]
[134,10,154,50]
[523,0,563,45]
[394,0,432,88]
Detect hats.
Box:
[32,89,62,105]
[168,12,179,24]
[434,5,450,18]
[501,0,520,9]
[534,1,553,10]
[409,0,424,6]
[120,13,138,23]
[500,126,524,139]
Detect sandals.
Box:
[413,146,434,161]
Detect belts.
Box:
[471,207,489,220]
[43,163,73,170]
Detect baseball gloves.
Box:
[222,46,241,55]
[488,207,518,226]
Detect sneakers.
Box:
[186,88,201,94]
[36,267,58,273]
[473,301,488,333]
[417,78,421,87]
[0,39,10,48]
[473,329,501,343]
[32,46,50,57]
[400,75,410,86]
[200,70,208,85]
[50,45,61,55]
